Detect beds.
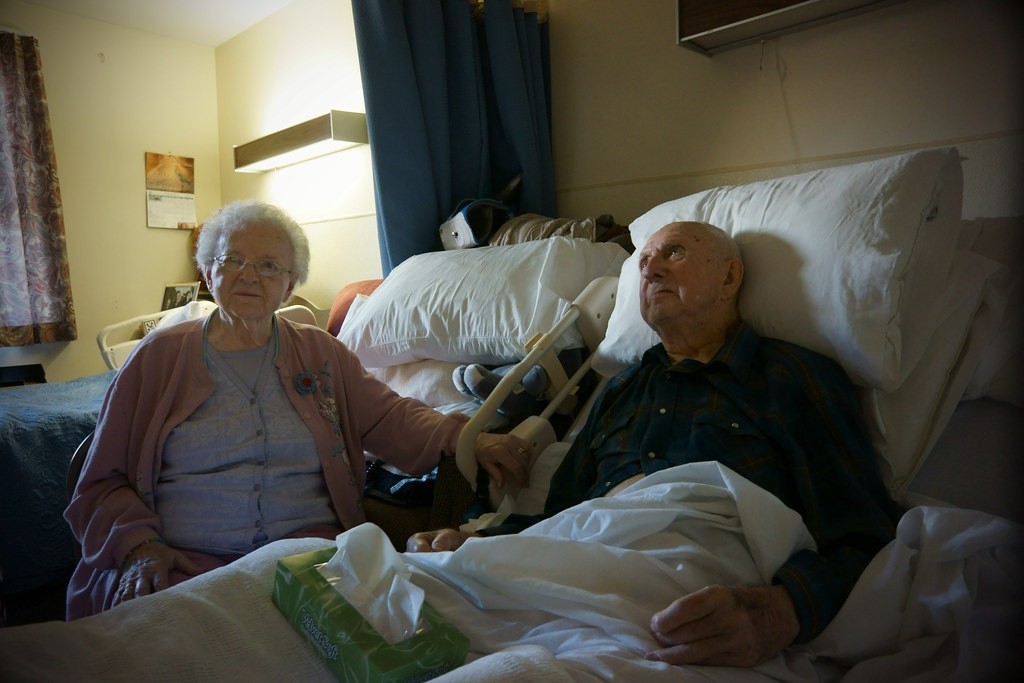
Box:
[3,147,1014,683]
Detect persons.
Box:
[62,201,533,621]
[408,219,898,669]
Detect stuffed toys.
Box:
[451,352,592,425]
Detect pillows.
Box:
[330,236,633,366]
[579,147,963,379]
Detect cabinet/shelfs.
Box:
[673,0,913,60]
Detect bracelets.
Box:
[119,537,166,575]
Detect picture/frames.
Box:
[159,281,201,312]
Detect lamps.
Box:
[229,109,369,177]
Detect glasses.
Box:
[213,253,292,278]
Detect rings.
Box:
[513,448,524,457]
